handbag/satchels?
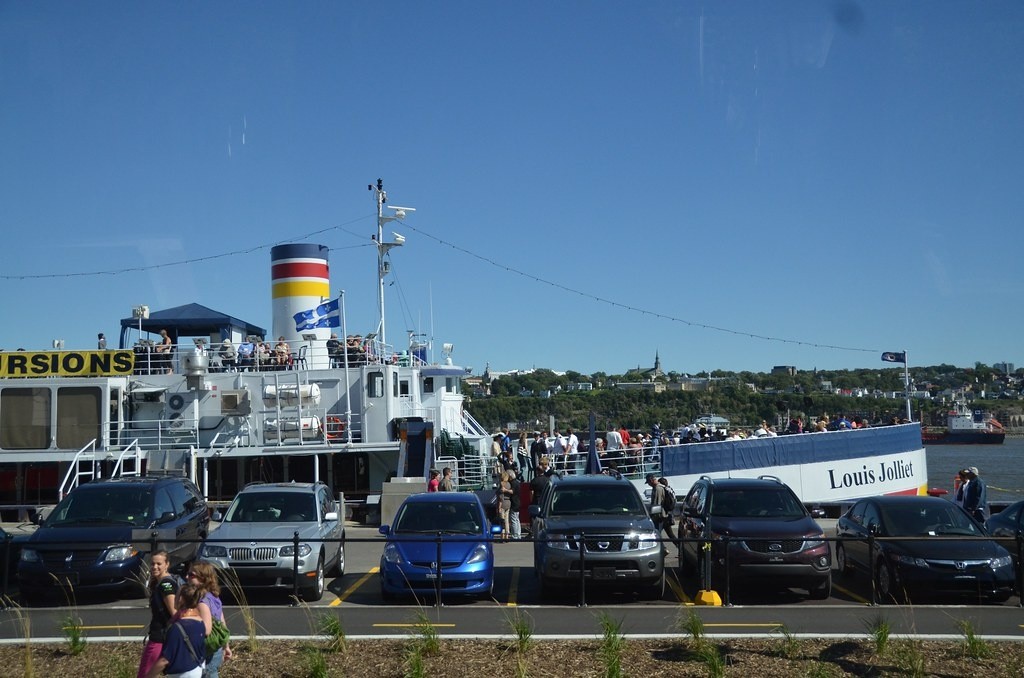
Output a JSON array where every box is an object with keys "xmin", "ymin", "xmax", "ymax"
[
  {"xmin": 202, "ymin": 597, "xmax": 230, "ymax": 660},
  {"xmin": 975, "ymin": 505, "xmax": 990, "ymax": 522}
]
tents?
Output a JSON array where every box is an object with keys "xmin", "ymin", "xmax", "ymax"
[{"xmin": 118, "ymin": 302, "xmax": 267, "ymax": 349}]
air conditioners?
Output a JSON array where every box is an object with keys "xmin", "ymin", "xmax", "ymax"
[{"xmin": 166, "ymin": 392, "xmax": 200, "ymax": 431}]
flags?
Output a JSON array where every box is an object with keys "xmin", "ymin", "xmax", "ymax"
[
  {"xmin": 292, "ymin": 298, "xmax": 340, "ymax": 332},
  {"xmin": 881, "ymin": 352, "xmax": 905, "ymax": 363}
]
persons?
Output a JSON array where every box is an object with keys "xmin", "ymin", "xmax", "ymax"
[
  {"xmin": 98, "ymin": 333, "xmax": 106, "ymax": 349},
  {"xmin": 491, "ymin": 428, "xmax": 579, "ymax": 542},
  {"xmin": 645, "ymin": 473, "xmax": 679, "ymax": 556},
  {"xmin": 427, "ymin": 470, "xmax": 441, "ymax": 492},
  {"xmin": 951, "ymin": 466, "xmax": 987, "ymax": 524},
  {"xmin": 580, "ymin": 419, "xmax": 777, "ymax": 477},
  {"xmin": 788, "ymin": 413, "xmax": 871, "ymax": 434},
  {"xmin": 888, "ymin": 416, "xmax": 911, "ymax": 425},
  {"xmin": 137, "ymin": 550, "xmax": 232, "ymax": 678},
  {"xmin": 156, "ymin": 329, "xmax": 175, "ymax": 372},
  {"xmin": 438, "ymin": 468, "xmax": 453, "ymax": 491},
  {"xmin": 217, "ymin": 335, "xmax": 290, "ymax": 371},
  {"xmin": 327, "ymin": 334, "xmax": 387, "ymax": 369}
]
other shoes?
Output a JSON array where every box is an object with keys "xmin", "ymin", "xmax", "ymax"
[{"xmin": 664, "ymin": 549, "xmax": 670, "ymax": 557}]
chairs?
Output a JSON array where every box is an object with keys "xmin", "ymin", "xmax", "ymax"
[
  {"xmin": 557, "ymin": 493, "xmax": 576, "ymax": 509},
  {"xmin": 205, "ymin": 348, "xmax": 217, "ymax": 372},
  {"xmin": 328, "ymin": 347, "xmax": 343, "ymax": 368},
  {"xmin": 434, "ymin": 428, "xmax": 481, "ymax": 483},
  {"xmin": 452, "ymin": 511, "xmax": 474, "ymax": 523},
  {"xmin": 291, "ymin": 345, "xmax": 309, "ymax": 370},
  {"xmin": 250, "ymin": 501, "xmax": 275, "ymax": 520}
]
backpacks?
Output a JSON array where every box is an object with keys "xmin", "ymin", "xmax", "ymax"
[{"xmin": 654, "ymin": 485, "xmax": 676, "ymax": 515}]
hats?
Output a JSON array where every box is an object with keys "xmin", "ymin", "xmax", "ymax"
[
  {"xmin": 964, "ymin": 466, "xmax": 979, "ymax": 476},
  {"xmin": 637, "ymin": 434, "xmax": 644, "ymax": 438},
  {"xmin": 222, "ymin": 338, "xmax": 231, "ymax": 348},
  {"xmin": 645, "ymin": 473, "xmax": 655, "ymax": 484},
  {"xmin": 532, "ymin": 430, "xmax": 541, "ymax": 436}
]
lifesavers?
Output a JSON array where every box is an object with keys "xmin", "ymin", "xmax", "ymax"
[{"xmin": 321, "ymin": 417, "xmax": 344, "ymax": 438}]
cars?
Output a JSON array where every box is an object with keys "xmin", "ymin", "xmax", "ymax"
[
  {"xmin": 378, "ymin": 492, "xmax": 501, "ymax": 602},
  {"xmin": 839, "ymin": 495, "xmax": 1024, "ymax": 604}
]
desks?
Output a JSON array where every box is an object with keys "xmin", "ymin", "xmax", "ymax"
[{"xmin": 134, "ymin": 351, "xmax": 168, "ymax": 374}]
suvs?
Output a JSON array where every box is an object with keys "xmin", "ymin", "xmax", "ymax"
[
  {"xmin": 18, "ymin": 473, "xmax": 214, "ymax": 602},
  {"xmin": 680, "ymin": 475, "xmax": 833, "ymax": 598},
  {"xmin": 533, "ymin": 476, "xmax": 667, "ymax": 599},
  {"xmin": 196, "ymin": 485, "xmax": 348, "ymax": 601}
]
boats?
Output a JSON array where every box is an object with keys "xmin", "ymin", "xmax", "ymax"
[
  {"xmin": 1, "ymin": 176, "xmax": 930, "ymax": 520},
  {"xmin": 920, "ymin": 401, "xmax": 1009, "ymax": 446}
]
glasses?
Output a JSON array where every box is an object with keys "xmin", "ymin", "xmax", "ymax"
[{"xmin": 186, "ymin": 571, "xmax": 205, "ymax": 583}]
